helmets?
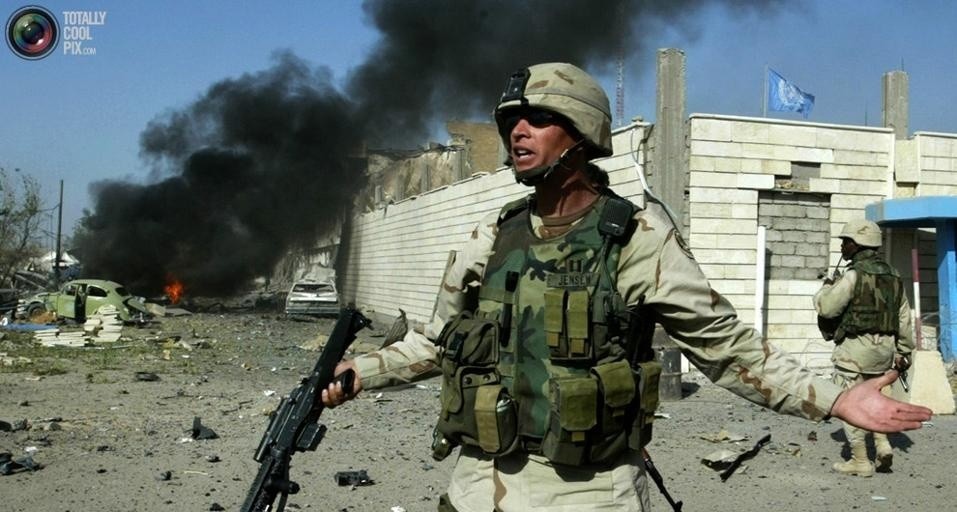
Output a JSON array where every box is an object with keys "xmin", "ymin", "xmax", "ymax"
[
  {"xmin": 837, "ymin": 219, "xmax": 883, "ymax": 247},
  {"xmin": 496, "ymin": 60, "xmax": 614, "ymax": 156}
]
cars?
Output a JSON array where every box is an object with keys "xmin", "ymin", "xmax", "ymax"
[
  {"xmin": 285, "ymin": 280, "xmax": 341, "ymax": 316},
  {"xmin": 14, "ymin": 279, "xmax": 154, "ymax": 324}
]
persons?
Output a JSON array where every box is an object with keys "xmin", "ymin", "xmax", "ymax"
[
  {"xmin": 813, "ymin": 218, "xmax": 913, "ymax": 478},
  {"xmin": 322, "ymin": 61, "xmax": 933, "ymax": 512}
]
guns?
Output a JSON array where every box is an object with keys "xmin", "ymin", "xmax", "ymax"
[
  {"xmin": 239, "ymin": 304, "xmax": 375, "ymax": 512},
  {"xmin": 642, "ymin": 447, "xmax": 683, "ymax": 512},
  {"xmin": 894, "ymin": 355, "xmax": 912, "ymax": 391}
]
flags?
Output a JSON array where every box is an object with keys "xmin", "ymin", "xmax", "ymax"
[{"xmin": 767, "ymin": 67, "xmax": 815, "ymax": 119}]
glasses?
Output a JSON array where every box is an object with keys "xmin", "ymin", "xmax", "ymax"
[{"xmin": 499, "ymin": 110, "xmax": 561, "ymax": 130}]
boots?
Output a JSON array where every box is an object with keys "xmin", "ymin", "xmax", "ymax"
[
  {"xmin": 874, "ymin": 430, "xmax": 894, "ymax": 473},
  {"xmin": 832, "ymin": 434, "xmax": 875, "ymax": 478}
]
